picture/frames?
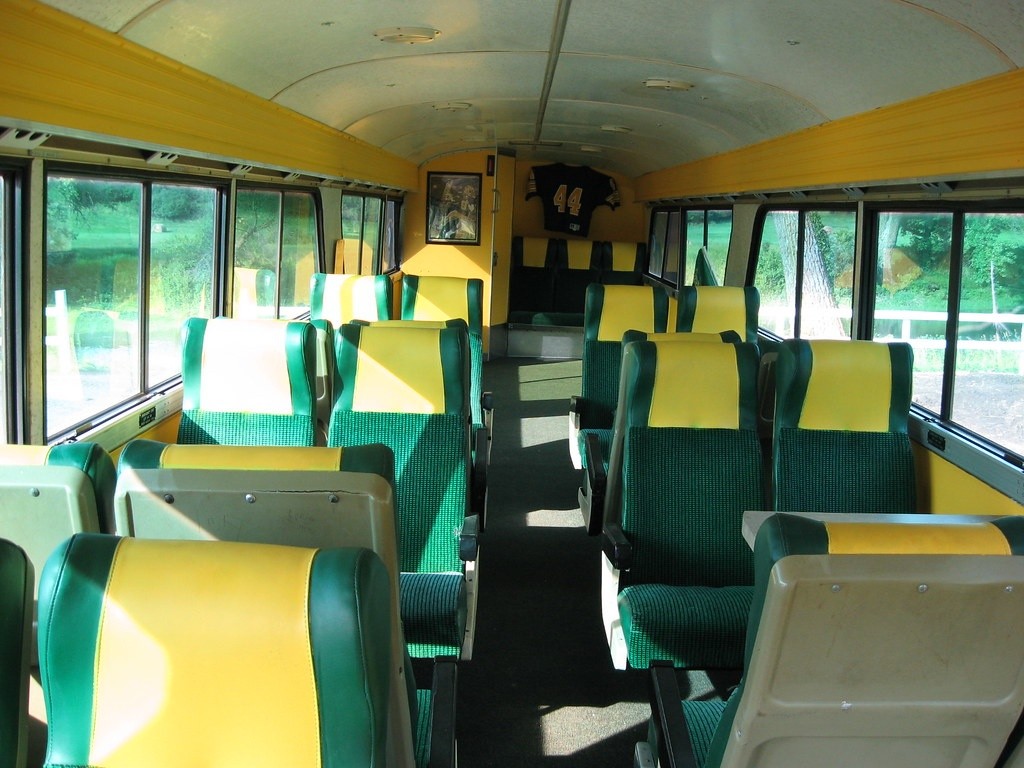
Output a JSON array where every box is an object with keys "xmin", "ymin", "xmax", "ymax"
[{"xmin": 426, "ymin": 171, "xmax": 482, "ymax": 246}]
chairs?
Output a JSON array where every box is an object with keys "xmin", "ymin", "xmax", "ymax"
[{"xmin": 0, "ymin": 236, "xmax": 1024, "ymax": 768}]
